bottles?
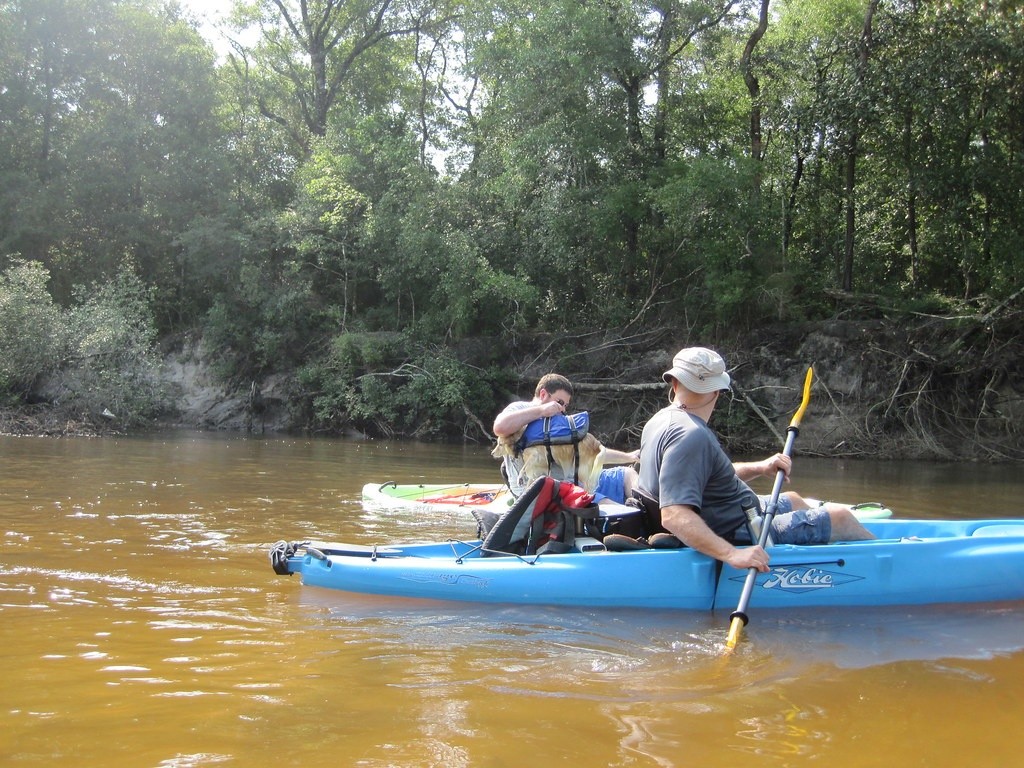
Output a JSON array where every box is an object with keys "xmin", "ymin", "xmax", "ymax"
[{"xmin": 745, "ymin": 507, "xmax": 773, "ymax": 546}]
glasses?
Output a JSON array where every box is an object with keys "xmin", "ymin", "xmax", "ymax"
[{"xmin": 549, "ymin": 393, "xmax": 568, "ymax": 408}]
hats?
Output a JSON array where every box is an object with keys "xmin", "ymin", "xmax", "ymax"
[{"xmin": 662, "ymin": 347, "xmax": 730, "ymax": 393}]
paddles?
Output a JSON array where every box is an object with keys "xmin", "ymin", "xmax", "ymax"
[{"xmin": 726, "ymin": 364, "xmax": 814, "ymax": 645}]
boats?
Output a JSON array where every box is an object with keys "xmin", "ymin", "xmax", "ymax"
[
  {"xmin": 359, "ymin": 482, "xmax": 893, "ymax": 518},
  {"xmin": 267, "ymin": 517, "xmax": 1023, "ymax": 612}
]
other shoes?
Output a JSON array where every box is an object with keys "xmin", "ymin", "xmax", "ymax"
[
  {"xmin": 603, "ymin": 534, "xmax": 651, "ymax": 550},
  {"xmin": 648, "ymin": 533, "xmax": 683, "ymax": 548}
]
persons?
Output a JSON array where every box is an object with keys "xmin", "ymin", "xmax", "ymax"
[
  {"xmin": 637, "ymin": 346, "xmax": 877, "ymax": 572},
  {"xmin": 493, "ymin": 373, "xmax": 640, "ymax": 503}
]
